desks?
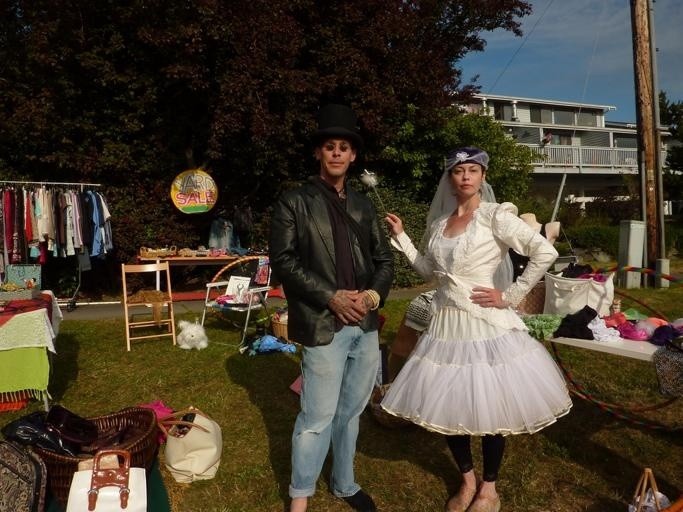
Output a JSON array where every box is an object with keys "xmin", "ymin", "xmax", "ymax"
[
  {"xmin": 135, "ymin": 247, "xmax": 268, "ymax": 308},
  {"xmin": 0, "ymin": 290, "xmax": 58, "ymax": 410},
  {"xmin": 520, "ymin": 310, "xmax": 682, "ymax": 371}
]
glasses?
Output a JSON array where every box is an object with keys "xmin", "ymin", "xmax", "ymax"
[{"xmin": 323, "ymin": 142, "xmax": 348, "ymax": 153}]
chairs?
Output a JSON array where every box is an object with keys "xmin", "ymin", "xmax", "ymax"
[
  {"xmin": 118, "ymin": 261, "xmax": 177, "ymax": 352},
  {"xmin": 200, "ymin": 254, "xmax": 272, "ymax": 347}
]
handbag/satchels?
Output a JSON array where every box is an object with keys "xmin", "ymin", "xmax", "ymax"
[
  {"xmin": 544, "ymin": 269, "xmax": 615, "ymax": 319},
  {"xmin": 164, "ymin": 405, "xmax": 222, "ymax": 484},
  {"xmin": 65, "ymin": 450, "xmax": 148, "ymax": 512}
]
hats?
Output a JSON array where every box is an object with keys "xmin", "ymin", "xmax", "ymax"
[{"xmin": 301, "ymin": 103, "xmax": 363, "ymax": 149}]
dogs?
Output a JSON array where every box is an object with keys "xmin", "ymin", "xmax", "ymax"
[{"xmin": 176, "ymin": 320, "xmax": 208, "ymax": 351}]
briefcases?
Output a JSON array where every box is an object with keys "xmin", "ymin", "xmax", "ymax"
[{"xmin": 0, "ymin": 263, "xmax": 42, "ymax": 301}]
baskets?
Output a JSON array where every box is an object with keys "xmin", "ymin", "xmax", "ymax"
[
  {"xmin": 515, "ymin": 280, "xmax": 545, "ymax": 315},
  {"xmin": 271, "ymin": 310, "xmax": 302, "ymax": 346},
  {"xmin": 6, "ymin": 408, "xmax": 158, "ymax": 512},
  {"xmin": 140, "ymin": 245, "xmax": 177, "ymax": 258},
  {"xmin": 368, "ymin": 384, "xmax": 413, "ymax": 427}
]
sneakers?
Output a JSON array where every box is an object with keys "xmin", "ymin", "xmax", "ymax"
[
  {"xmin": 445, "ymin": 481, "xmax": 479, "ymax": 511},
  {"xmin": 467, "ymin": 483, "xmax": 501, "ymax": 512}
]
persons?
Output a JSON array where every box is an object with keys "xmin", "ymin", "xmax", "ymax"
[
  {"xmin": 268, "ymin": 136, "xmax": 395, "ymax": 512},
  {"xmin": 379, "ymin": 146, "xmax": 573, "ymax": 512}
]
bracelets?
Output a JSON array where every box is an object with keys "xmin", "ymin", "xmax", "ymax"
[{"xmin": 365, "ymin": 289, "xmax": 381, "ymax": 311}]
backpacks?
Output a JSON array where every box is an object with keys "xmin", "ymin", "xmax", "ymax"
[{"xmin": 0, "ymin": 438, "xmax": 48, "ymax": 512}]
